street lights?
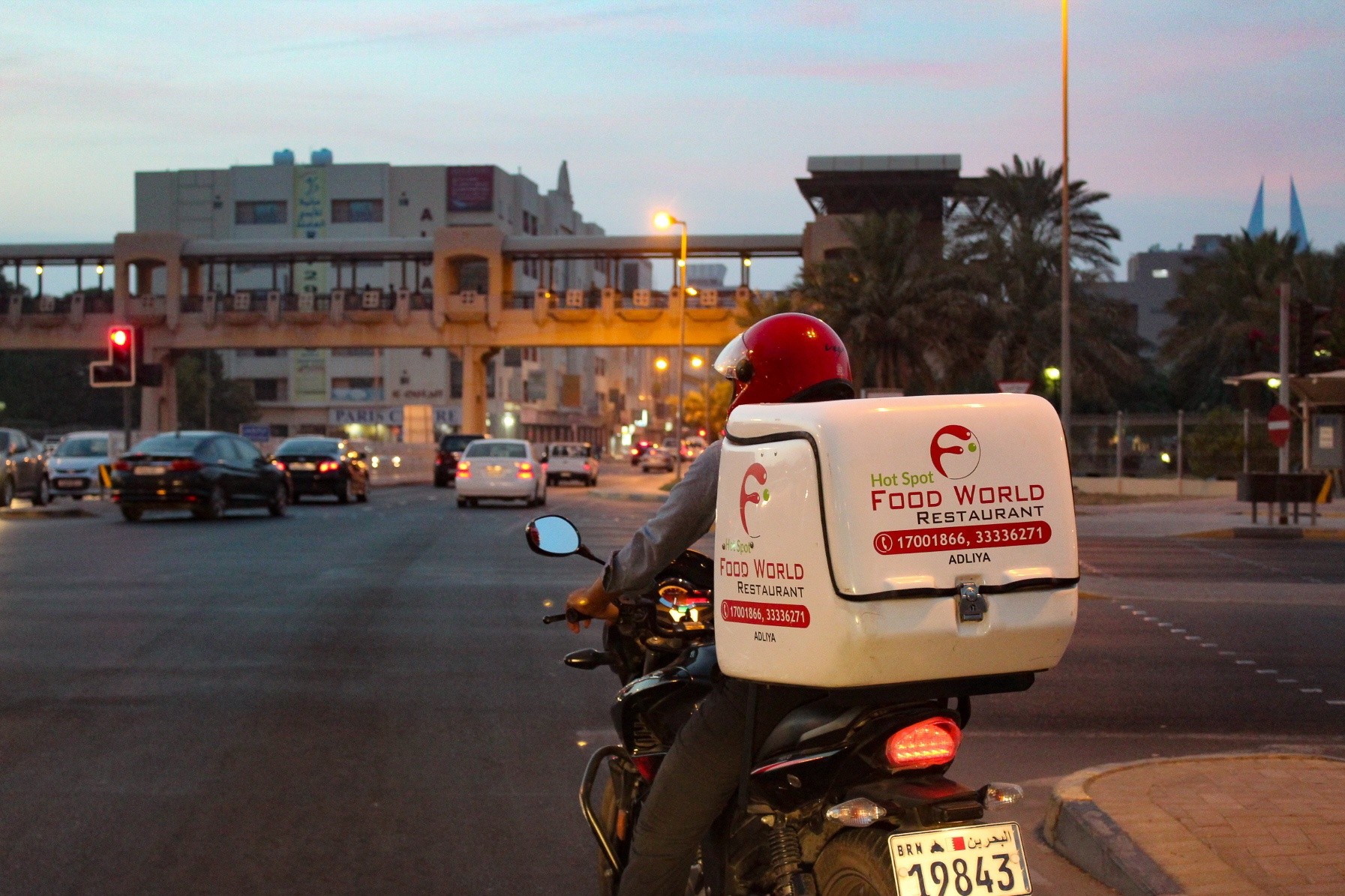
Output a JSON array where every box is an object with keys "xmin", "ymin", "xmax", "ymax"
[{"xmin": 654, "ymin": 213, "xmax": 682, "ymax": 483}]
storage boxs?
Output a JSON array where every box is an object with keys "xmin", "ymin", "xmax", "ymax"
[{"xmin": 709, "ymin": 395, "xmax": 1081, "ymax": 689}]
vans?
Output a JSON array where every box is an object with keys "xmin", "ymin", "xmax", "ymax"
[
  {"xmin": 433, "ymin": 434, "xmax": 486, "ymax": 488},
  {"xmin": 541, "ymin": 442, "xmax": 600, "ymax": 488}
]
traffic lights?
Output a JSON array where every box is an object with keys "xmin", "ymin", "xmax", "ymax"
[{"xmin": 90, "ymin": 325, "xmax": 135, "ymax": 388}]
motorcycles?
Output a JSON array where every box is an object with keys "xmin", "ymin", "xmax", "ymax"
[{"xmin": 519, "ymin": 387, "xmax": 1082, "ymax": 896}]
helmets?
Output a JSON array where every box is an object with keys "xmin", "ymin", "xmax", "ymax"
[{"xmin": 713, "ymin": 311, "xmax": 856, "ymax": 424}]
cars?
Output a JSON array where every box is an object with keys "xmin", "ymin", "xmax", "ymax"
[
  {"xmin": 43, "ymin": 432, "xmax": 122, "ymax": 502},
  {"xmin": 110, "ymin": 430, "xmax": 294, "ymax": 520},
  {"xmin": 451, "ymin": 438, "xmax": 550, "ymax": 508},
  {"xmin": 630, "ymin": 435, "xmax": 707, "ymax": 474},
  {"xmin": 272, "ymin": 435, "xmax": 370, "ymax": 506},
  {"xmin": 0, "ymin": 428, "xmax": 49, "ymax": 510}
]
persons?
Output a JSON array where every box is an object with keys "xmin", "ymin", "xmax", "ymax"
[{"xmin": 560, "ymin": 311, "xmax": 884, "ymax": 896}]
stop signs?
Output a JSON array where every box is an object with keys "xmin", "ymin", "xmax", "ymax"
[{"xmin": 1266, "ymin": 404, "xmax": 1290, "ymax": 448}]
